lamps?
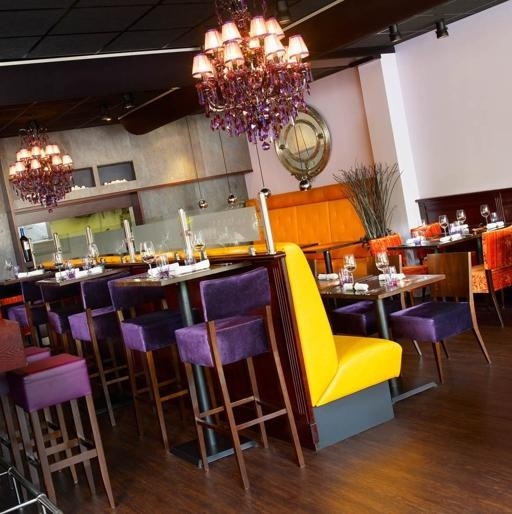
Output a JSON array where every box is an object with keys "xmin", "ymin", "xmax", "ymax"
[
  {"xmin": 190, "ymin": 1, "xmax": 316, "ymax": 156},
  {"xmin": 5, "ymin": 122, "xmax": 77, "ymax": 214}
]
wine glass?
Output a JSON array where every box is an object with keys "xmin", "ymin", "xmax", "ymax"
[
  {"xmin": 374, "ymin": 251, "xmax": 390, "ymax": 286},
  {"xmin": 439, "ymin": 205, "xmax": 490, "ymax": 238},
  {"xmin": 139, "ymin": 241, "xmax": 156, "ymax": 279},
  {"xmin": 344, "ymin": 254, "xmax": 357, "ymax": 283},
  {"xmin": 190, "ymin": 231, "xmax": 205, "ymax": 262},
  {"xmin": 52, "ymin": 251, "xmax": 64, "ymax": 281},
  {"xmin": 88, "ymin": 243, "xmax": 100, "ymax": 269}
]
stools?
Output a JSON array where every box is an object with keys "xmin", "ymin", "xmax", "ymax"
[
  {"xmin": 174, "ymin": 265, "xmax": 308, "ymax": 490},
  {"xmin": 1, "ymin": 343, "xmax": 79, "ymax": 501},
  {"xmin": 9, "ymin": 352, "xmax": 115, "ymax": 507},
  {"xmin": 1, "ymin": 267, "xmax": 156, "ymax": 428},
  {"xmin": 108, "ymin": 270, "xmax": 220, "ymax": 452}
]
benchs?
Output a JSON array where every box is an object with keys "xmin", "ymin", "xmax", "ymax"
[
  {"xmin": 41, "ymin": 240, "xmax": 402, "ymax": 452},
  {"xmin": 241, "ymin": 178, "xmax": 378, "ymax": 285}
]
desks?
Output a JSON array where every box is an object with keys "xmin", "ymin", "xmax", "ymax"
[{"xmin": 114, "ymin": 259, "xmax": 257, "ymax": 470}]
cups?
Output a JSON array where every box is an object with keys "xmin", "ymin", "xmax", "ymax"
[
  {"xmin": 383, "ymin": 266, "xmax": 400, "ymax": 292},
  {"xmin": 339, "ymin": 267, "xmax": 354, "ymax": 288},
  {"xmin": 156, "ymin": 255, "xmax": 168, "ymax": 274},
  {"xmin": 490, "ymin": 212, "xmax": 498, "ymax": 224},
  {"xmin": 64, "ymin": 256, "xmax": 93, "ymax": 278}
]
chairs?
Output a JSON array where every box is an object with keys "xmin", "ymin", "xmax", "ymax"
[{"xmin": 326, "ymin": 220, "xmax": 511, "ymax": 387}]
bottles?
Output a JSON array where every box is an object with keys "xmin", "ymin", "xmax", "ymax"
[{"xmin": 20, "ymin": 228, "xmax": 32, "ymax": 263}]
